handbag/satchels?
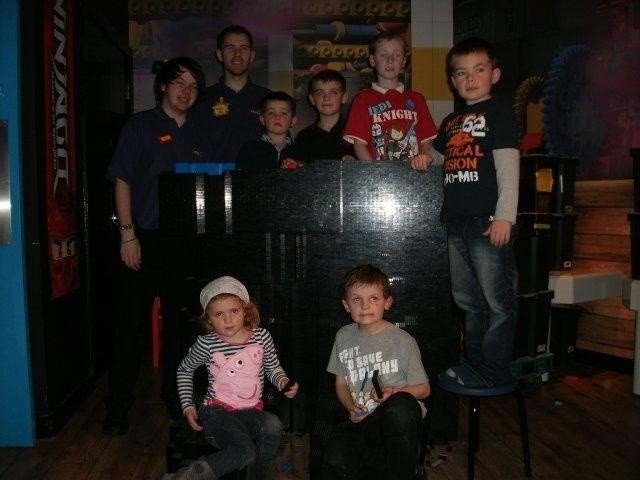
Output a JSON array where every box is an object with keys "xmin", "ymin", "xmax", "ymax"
[
  {"xmin": 119, "ymin": 223, "xmax": 137, "ymax": 232},
  {"xmin": 120, "ymin": 236, "xmax": 138, "ymax": 247}
]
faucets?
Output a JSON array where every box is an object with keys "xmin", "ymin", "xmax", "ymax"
[{"xmin": 443, "ymin": 361, "xmax": 505, "ymax": 387}]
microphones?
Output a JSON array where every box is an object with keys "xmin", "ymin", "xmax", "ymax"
[{"xmin": 423, "ymin": 365, "xmax": 533, "ymax": 480}]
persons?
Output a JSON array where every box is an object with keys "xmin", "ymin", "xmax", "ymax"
[
  {"xmin": 101, "ymin": 57, "xmax": 214, "ymax": 436},
  {"xmin": 325, "ymin": 264, "xmax": 432, "ymax": 480},
  {"xmin": 280, "ymin": 70, "xmax": 356, "ymax": 161},
  {"xmin": 339, "ymin": 31, "xmax": 439, "ymax": 161},
  {"xmin": 234, "ymin": 91, "xmax": 298, "ymax": 163},
  {"xmin": 170, "ymin": 275, "xmax": 299, "ymax": 480},
  {"xmin": 409, "ymin": 40, "xmax": 521, "ymax": 390},
  {"xmin": 188, "ymin": 25, "xmax": 272, "ymax": 163}
]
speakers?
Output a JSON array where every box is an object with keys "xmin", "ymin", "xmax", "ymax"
[
  {"xmin": 165, "ymin": 456, "xmax": 216, "ymax": 480},
  {"xmin": 252, "ymin": 459, "xmax": 281, "ymax": 478}
]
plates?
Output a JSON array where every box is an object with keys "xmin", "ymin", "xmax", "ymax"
[{"xmin": 504, "ymin": 151, "xmax": 580, "ymax": 382}]
